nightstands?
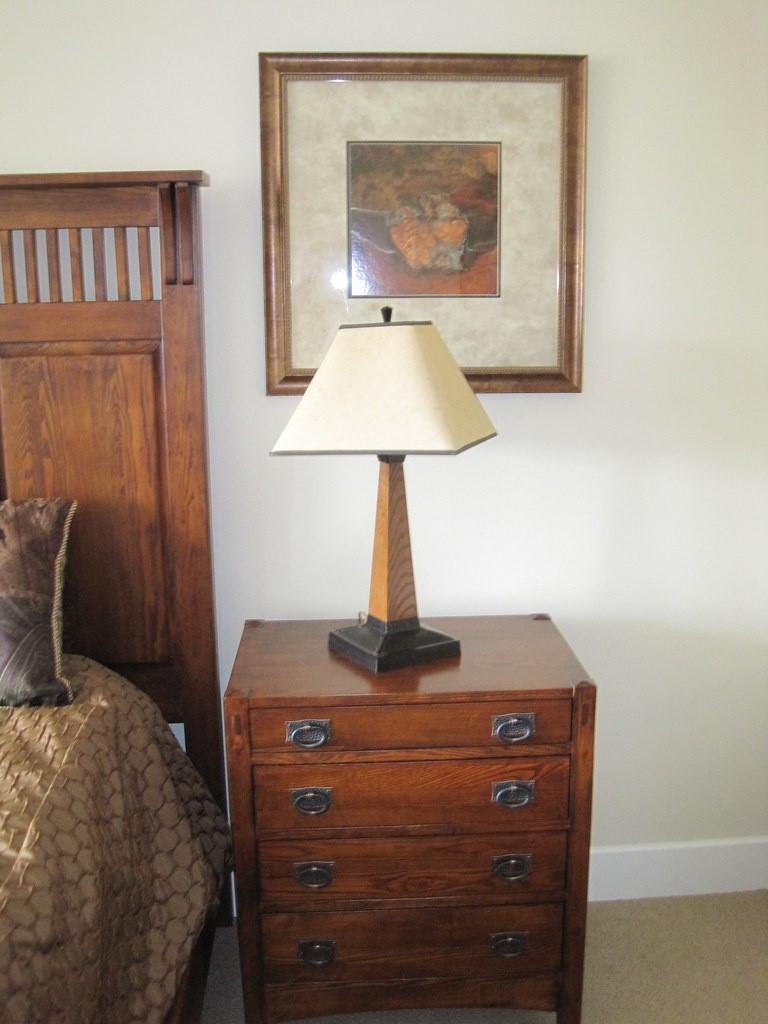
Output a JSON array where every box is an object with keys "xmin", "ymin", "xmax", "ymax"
[{"xmin": 223, "ymin": 614, "xmax": 598, "ymax": 1024}]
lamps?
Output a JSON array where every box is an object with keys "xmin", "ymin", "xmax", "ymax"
[{"xmin": 267, "ymin": 305, "xmax": 497, "ymax": 674}]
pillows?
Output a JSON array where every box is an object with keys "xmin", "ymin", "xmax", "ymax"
[{"xmin": 0, "ymin": 496, "xmax": 78, "ymax": 709}]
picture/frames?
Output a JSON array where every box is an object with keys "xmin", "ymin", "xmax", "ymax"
[{"xmin": 257, "ymin": 51, "xmax": 589, "ymax": 396}]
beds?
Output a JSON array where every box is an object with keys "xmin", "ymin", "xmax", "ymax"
[{"xmin": 0, "ymin": 170, "xmax": 234, "ymax": 1024}]
